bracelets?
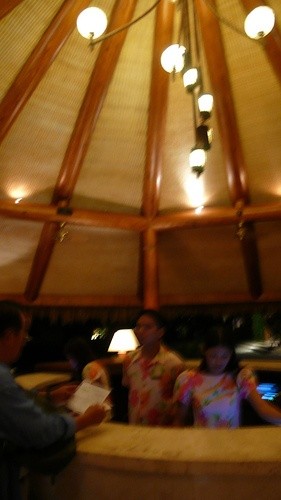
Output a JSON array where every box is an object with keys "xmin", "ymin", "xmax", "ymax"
[{"xmin": 44, "ymin": 388, "xmax": 54, "ymax": 409}]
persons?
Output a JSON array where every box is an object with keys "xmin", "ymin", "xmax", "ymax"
[
  {"xmin": 121, "ymin": 308, "xmax": 191, "ymax": 429},
  {"xmin": 0, "ymin": 298, "xmax": 108, "ymax": 500},
  {"xmin": 170, "ymin": 336, "xmax": 281, "ymax": 431}
]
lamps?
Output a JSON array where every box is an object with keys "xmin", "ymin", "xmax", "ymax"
[
  {"xmin": 106, "ymin": 328, "xmax": 140, "ymax": 356},
  {"xmin": 75, "ymin": 0, "xmax": 275, "ymax": 182}
]
